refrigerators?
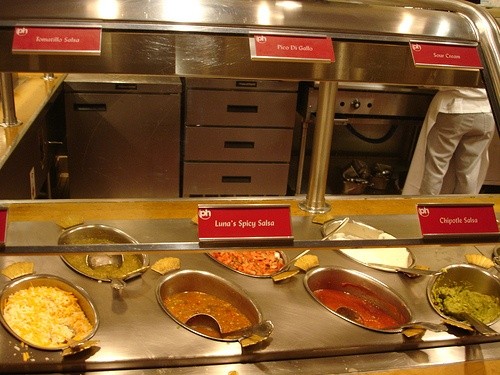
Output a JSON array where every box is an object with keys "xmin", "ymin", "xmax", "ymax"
[{"xmin": 62, "ymin": 71, "xmax": 179, "ymax": 198}]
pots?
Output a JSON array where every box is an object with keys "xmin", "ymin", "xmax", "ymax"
[
  {"xmin": 205, "ymin": 248, "xmax": 289, "ymax": 277},
  {"xmin": 0, "ymin": 273, "xmax": 99, "ymax": 351},
  {"xmin": 154, "ymin": 268, "xmax": 262, "ymax": 342},
  {"xmin": 59, "ymin": 224, "xmax": 149, "ymax": 282},
  {"xmin": 321, "ymin": 217, "xmax": 415, "ymax": 274},
  {"xmin": 426, "ymin": 263, "xmax": 500, "ymax": 328},
  {"xmin": 303, "ymin": 265, "xmax": 413, "ymax": 334}
]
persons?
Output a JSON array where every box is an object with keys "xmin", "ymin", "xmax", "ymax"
[{"xmin": 401, "ymin": 0, "xmax": 496, "ymax": 196}]
